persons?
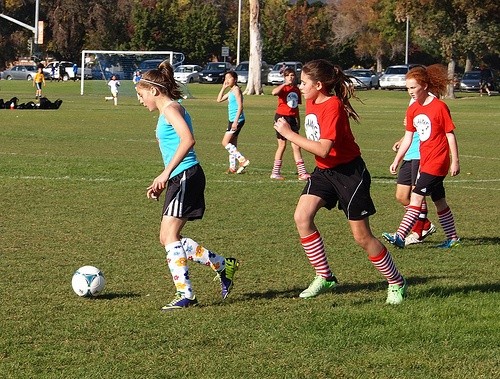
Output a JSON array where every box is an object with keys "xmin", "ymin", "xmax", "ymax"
[
  {"xmin": 133, "ymin": 70, "xmax": 142, "ymax": 84},
  {"xmin": 217, "ymin": 71, "xmax": 250, "ymax": 175},
  {"xmin": 381, "ymin": 63, "xmax": 461, "ymax": 248},
  {"xmin": 33, "ymin": 67, "xmax": 45, "ymax": 102},
  {"xmin": 136, "ymin": 60, "xmax": 241, "ymax": 309},
  {"xmin": 479, "ymin": 65, "xmax": 492, "ymax": 97},
  {"xmin": 393, "ymin": 64, "xmax": 436, "ymax": 245},
  {"xmin": 73, "ymin": 64, "xmax": 79, "ymax": 82},
  {"xmin": 272, "ymin": 68, "xmax": 312, "ymax": 181},
  {"xmin": 50, "ymin": 65, "xmax": 65, "ymax": 82},
  {"xmin": 105, "ymin": 75, "xmax": 120, "ymax": 106},
  {"xmin": 274, "ymin": 59, "xmax": 406, "ymax": 304}
]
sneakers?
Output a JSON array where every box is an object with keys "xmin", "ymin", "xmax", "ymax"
[
  {"xmin": 382, "ymin": 232, "xmax": 405, "ymax": 249},
  {"xmin": 213, "ymin": 258, "xmax": 240, "ymax": 300},
  {"xmin": 298, "ymin": 173, "xmax": 311, "ymax": 181},
  {"xmin": 161, "ymin": 292, "xmax": 199, "ymax": 310},
  {"xmin": 224, "ymin": 167, "xmax": 237, "ymax": 175},
  {"xmin": 422, "ymin": 223, "xmax": 437, "ymax": 239},
  {"xmin": 405, "ymin": 232, "xmax": 422, "ymax": 246},
  {"xmin": 386, "ymin": 276, "xmax": 408, "ymax": 305},
  {"xmin": 299, "ymin": 272, "xmax": 338, "ymax": 300},
  {"xmin": 270, "ymin": 173, "xmax": 285, "ymax": 180},
  {"xmin": 237, "ymin": 159, "xmax": 250, "ymax": 174},
  {"xmin": 436, "ymin": 237, "xmax": 461, "ymax": 249}
]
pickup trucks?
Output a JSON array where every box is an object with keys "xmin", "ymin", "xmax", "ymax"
[{"xmin": 42, "ymin": 60, "xmax": 93, "ymax": 80}]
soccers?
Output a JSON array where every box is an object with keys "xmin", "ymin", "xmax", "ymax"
[{"xmin": 71, "ymin": 265, "xmax": 107, "ymax": 297}]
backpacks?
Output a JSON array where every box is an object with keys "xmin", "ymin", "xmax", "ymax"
[{"xmin": 0, "ymin": 97, "xmax": 62, "ymax": 109}]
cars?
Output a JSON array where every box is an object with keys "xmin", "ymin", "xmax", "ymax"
[
  {"xmin": 378, "ymin": 64, "xmax": 428, "ymax": 90},
  {"xmin": 229, "ymin": 61, "xmax": 271, "ymax": 84},
  {"xmin": 266, "ymin": 61, "xmax": 305, "ymax": 85},
  {"xmin": 460, "ymin": 71, "xmax": 495, "ymax": 93},
  {"xmin": 198, "ymin": 62, "xmax": 233, "ymax": 84},
  {"xmin": 345, "ymin": 69, "xmax": 381, "ymax": 90},
  {"xmin": 2, "ymin": 64, "xmax": 51, "ymax": 82},
  {"xmin": 173, "ymin": 64, "xmax": 204, "ymax": 84},
  {"xmin": 131, "ymin": 59, "xmax": 165, "ymax": 80}
]
